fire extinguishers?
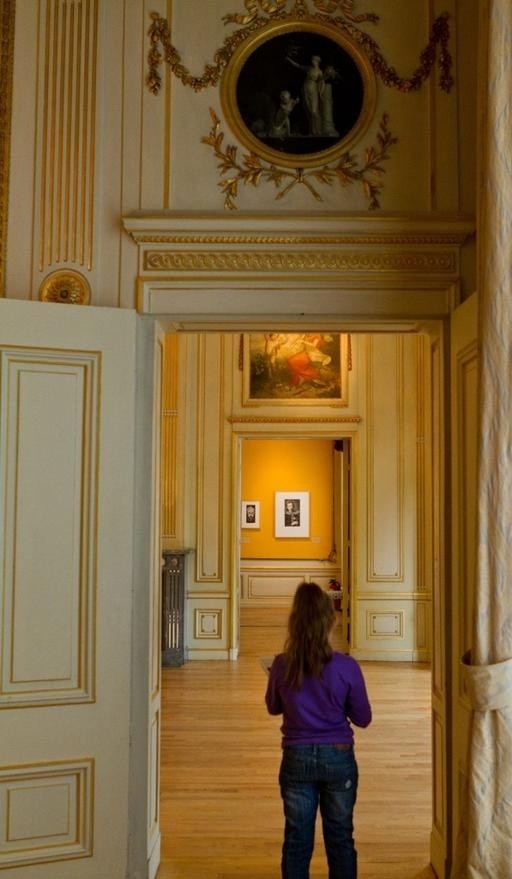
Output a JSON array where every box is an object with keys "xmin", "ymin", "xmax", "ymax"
[{"xmin": 328, "ymin": 579, "xmax": 341, "ymax": 612}]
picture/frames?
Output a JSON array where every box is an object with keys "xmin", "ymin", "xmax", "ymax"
[
  {"xmin": 274, "ymin": 491, "xmax": 310, "ymax": 538},
  {"xmin": 240, "ymin": 331, "xmax": 348, "ymax": 409},
  {"xmin": 240, "ymin": 501, "xmax": 260, "ymax": 529},
  {"xmin": 218, "ymin": 17, "xmax": 379, "ymax": 170}
]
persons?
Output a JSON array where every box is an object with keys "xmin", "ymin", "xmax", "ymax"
[
  {"xmin": 269, "ymin": 89, "xmax": 298, "ymax": 138},
  {"xmin": 286, "ymin": 53, "xmax": 326, "ymax": 135},
  {"xmin": 288, "ymin": 331, "xmax": 335, "ymax": 387},
  {"xmin": 285, "ymin": 502, "xmax": 299, "ymax": 526},
  {"xmin": 264, "ymin": 583, "xmax": 373, "ymax": 879},
  {"xmin": 263, "ymin": 333, "xmax": 286, "ymax": 384}
]
